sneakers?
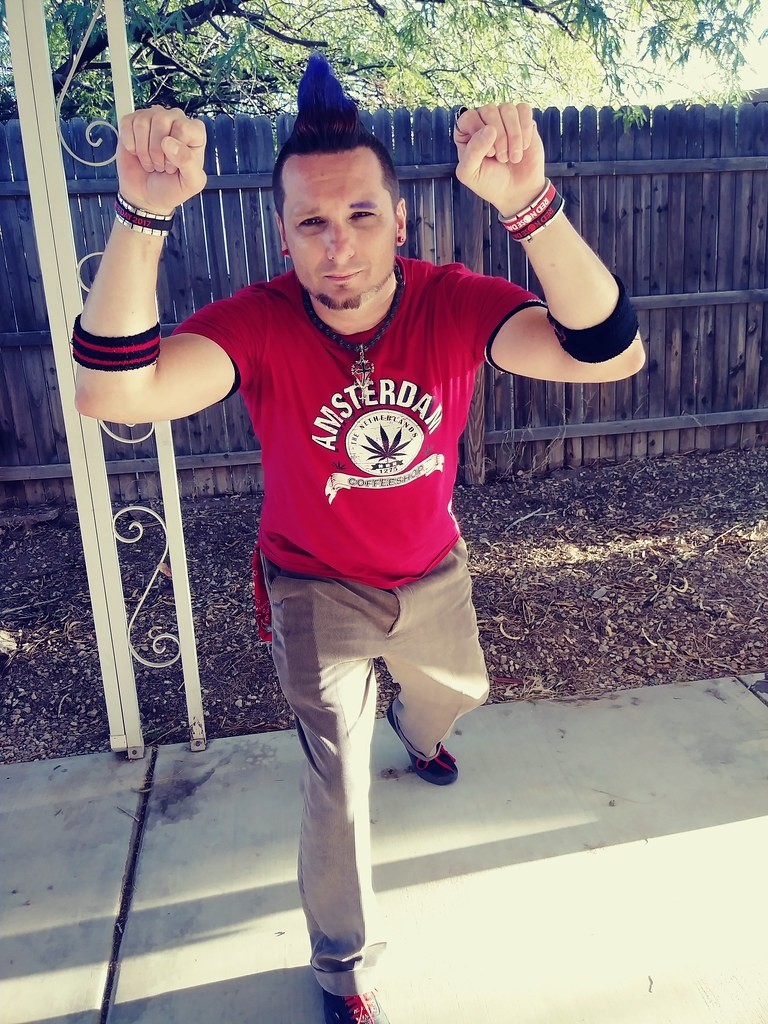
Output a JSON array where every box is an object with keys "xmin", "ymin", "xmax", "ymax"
[
  {"xmin": 387, "ymin": 705, "xmax": 458, "ymax": 785},
  {"xmin": 321, "ymin": 988, "xmax": 390, "ymax": 1024}
]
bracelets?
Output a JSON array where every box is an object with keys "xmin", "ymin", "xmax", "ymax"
[
  {"xmin": 70, "ymin": 312, "xmax": 161, "ymax": 372},
  {"xmin": 495, "ymin": 177, "xmax": 566, "ymax": 246},
  {"xmin": 111, "ymin": 191, "xmax": 178, "ymax": 241},
  {"xmin": 546, "ymin": 275, "xmax": 640, "ymax": 364}
]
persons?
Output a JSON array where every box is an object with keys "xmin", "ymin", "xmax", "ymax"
[{"xmin": 65, "ymin": 46, "xmax": 647, "ymax": 1023}]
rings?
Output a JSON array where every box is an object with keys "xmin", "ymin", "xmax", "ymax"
[{"xmin": 453, "ymin": 105, "xmax": 472, "ymax": 135}]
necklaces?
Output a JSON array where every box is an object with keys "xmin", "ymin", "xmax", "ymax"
[{"xmin": 300, "ymin": 260, "xmax": 403, "ymax": 390}]
